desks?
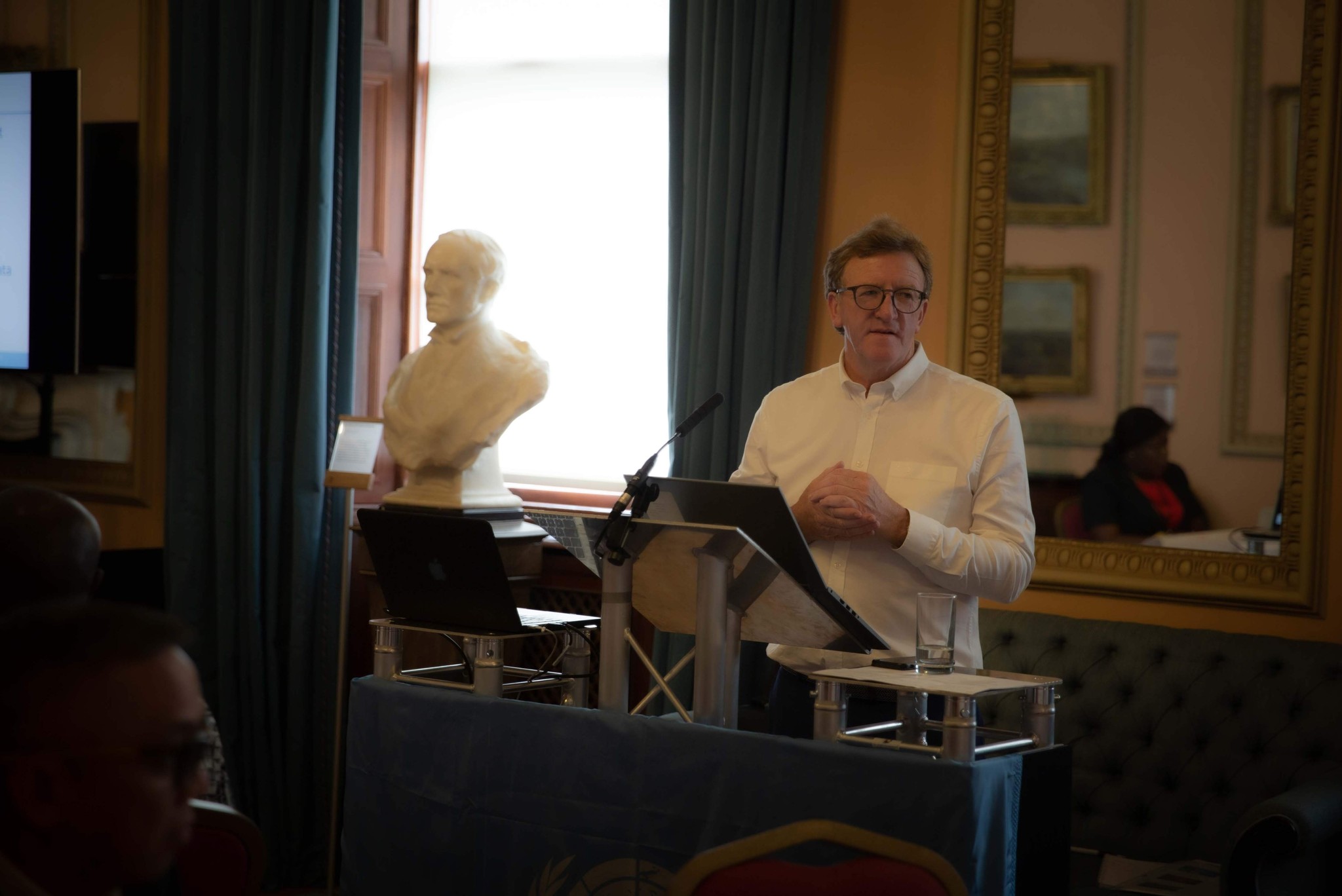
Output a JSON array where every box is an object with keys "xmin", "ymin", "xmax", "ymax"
[{"xmin": 340, "ymin": 676, "xmax": 1073, "ymax": 896}]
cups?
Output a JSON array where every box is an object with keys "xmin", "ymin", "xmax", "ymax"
[{"xmin": 916, "ymin": 593, "xmax": 958, "ymax": 675}]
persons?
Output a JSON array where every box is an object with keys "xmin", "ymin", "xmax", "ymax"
[
  {"xmin": 0, "ymin": 490, "xmax": 221, "ymax": 896},
  {"xmin": 381, "ymin": 229, "xmax": 549, "ymax": 512},
  {"xmin": 1080, "ymin": 405, "xmax": 1215, "ymax": 543},
  {"xmin": 728, "ymin": 214, "xmax": 1035, "ymax": 745}
]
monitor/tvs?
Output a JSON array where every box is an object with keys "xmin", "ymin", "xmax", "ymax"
[{"xmin": 0, "ymin": 68, "xmax": 85, "ymax": 374}]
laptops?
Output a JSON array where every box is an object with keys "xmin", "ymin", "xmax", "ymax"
[
  {"xmin": 356, "ymin": 508, "xmax": 601, "ymax": 635},
  {"xmin": 624, "ymin": 474, "xmax": 894, "ymax": 653},
  {"xmin": 1242, "ymin": 488, "xmax": 1284, "ymax": 540}
]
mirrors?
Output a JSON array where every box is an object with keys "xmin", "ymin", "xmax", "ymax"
[
  {"xmin": 952, "ymin": 0, "xmax": 1341, "ymax": 622},
  {"xmin": 43, "ymin": 1, "xmax": 162, "ymax": 508}
]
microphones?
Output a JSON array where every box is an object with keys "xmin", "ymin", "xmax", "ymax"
[{"xmin": 606, "ymin": 392, "xmax": 725, "ymax": 522}]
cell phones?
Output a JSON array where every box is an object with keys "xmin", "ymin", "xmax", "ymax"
[{"xmin": 871, "ymin": 656, "xmax": 917, "ymax": 670}]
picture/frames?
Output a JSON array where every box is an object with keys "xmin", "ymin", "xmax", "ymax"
[
  {"xmin": 998, "ymin": 266, "xmax": 1091, "ymax": 397},
  {"xmin": 1004, "ymin": 59, "xmax": 1112, "ymax": 227},
  {"xmin": 1267, "ymin": 84, "xmax": 1299, "ymax": 227}
]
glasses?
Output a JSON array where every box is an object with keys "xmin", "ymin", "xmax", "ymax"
[
  {"xmin": 835, "ymin": 285, "xmax": 928, "ymax": 313},
  {"xmin": 9, "ymin": 725, "xmax": 225, "ymax": 784}
]
